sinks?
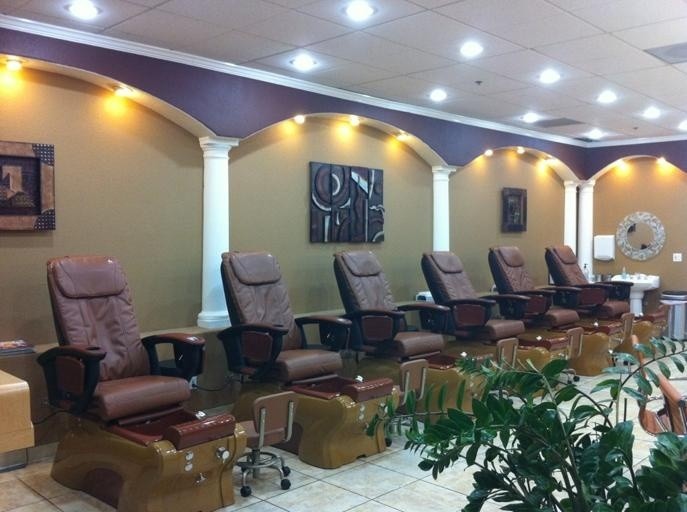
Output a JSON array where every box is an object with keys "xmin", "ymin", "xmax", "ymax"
[{"xmin": 610, "ymin": 274, "xmax": 660, "ymax": 291}]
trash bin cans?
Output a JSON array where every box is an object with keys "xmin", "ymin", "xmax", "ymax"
[{"xmin": 659, "ymin": 290, "xmax": 687, "ymax": 341}]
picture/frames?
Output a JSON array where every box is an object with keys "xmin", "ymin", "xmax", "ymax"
[
  {"xmin": 502, "ymin": 187, "xmax": 528, "ymax": 233},
  {"xmin": 0, "ymin": 141, "xmax": 56, "ymax": 231}
]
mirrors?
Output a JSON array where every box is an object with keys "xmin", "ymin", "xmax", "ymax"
[{"xmin": 616, "ymin": 212, "xmax": 666, "ymax": 261}]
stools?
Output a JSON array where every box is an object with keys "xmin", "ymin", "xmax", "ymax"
[
  {"xmin": 385, "ymin": 358, "xmax": 430, "ymax": 448},
  {"xmin": 556, "ymin": 327, "xmax": 584, "ymax": 385},
  {"xmin": 235, "ymin": 390, "xmax": 296, "ymax": 497}
]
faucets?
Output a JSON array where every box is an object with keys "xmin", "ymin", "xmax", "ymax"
[{"xmin": 635, "ymin": 272, "xmax": 640, "ymax": 280}]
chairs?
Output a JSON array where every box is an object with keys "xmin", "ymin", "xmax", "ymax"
[
  {"xmin": 216, "ymin": 250, "xmax": 399, "ymax": 470},
  {"xmin": 420, "ymin": 251, "xmax": 559, "ymax": 397},
  {"xmin": 39, "ymin": 254, "xmax": 248, "ymax": 512},
  {"xmin": 657, "ymin": 375, "xmax": 687, "ymax": 438},
  {"xmin": 487, "ymin": 246, "xmax": 668, "ymax": 378},
  {"xmin": 332, "ymin": 249, "xmax": 490, "ymax": 425}
]
